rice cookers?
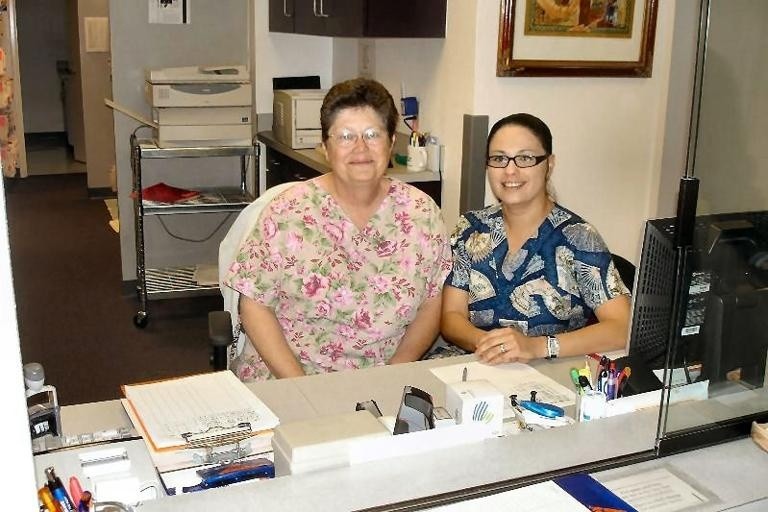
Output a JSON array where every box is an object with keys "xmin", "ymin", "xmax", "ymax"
[{"xmin": 196, "ymin": 459, "xmax": 276, "ymax": 490}]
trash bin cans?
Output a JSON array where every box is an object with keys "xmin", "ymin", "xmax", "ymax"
[{"xmin": 543, "ymin": 334, "xmax": 558, "ymax": 360}]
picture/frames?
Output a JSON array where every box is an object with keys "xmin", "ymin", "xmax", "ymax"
[{"xmin": 33, "ymin": 347, "xmax": 765, "ymax": 511}]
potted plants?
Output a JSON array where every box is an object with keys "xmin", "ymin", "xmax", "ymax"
[{"xmin": 494, "ymin": 0, "xmax": 659, "ymax": 80}]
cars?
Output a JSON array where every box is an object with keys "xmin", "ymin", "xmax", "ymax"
[{"xmin": 405, "ymin": 144, "xmax": 428, "ymax": 172}]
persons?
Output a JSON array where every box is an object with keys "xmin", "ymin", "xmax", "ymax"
[
  {"xmin": 224, "ymin": 76, "xmax": 452, "ymax": 383},
  {"xmin": 422, "ymin": 113, "xmax": 632, "ymax": 367}
]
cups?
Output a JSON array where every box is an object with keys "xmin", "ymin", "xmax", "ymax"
[
  {"xmin": 326, "ymin": 126, "xmax": 389, "ymax": 148},
  {"xmin": 485, "ymin": 144, "xmax": 549, "ymax": 169}
]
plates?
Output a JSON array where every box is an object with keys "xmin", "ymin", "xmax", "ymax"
[
  {"xmin": 258, "ymin": 128, "xmax": 440, "ymax": 215},
  {"xmin": 261, "ymin": 0, "xmax": 449, "ymax": 40}
]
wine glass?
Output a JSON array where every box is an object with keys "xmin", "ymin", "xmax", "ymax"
[{"xmin": 500, "ymin": 343, "xmax": 508, "ymax": 354}]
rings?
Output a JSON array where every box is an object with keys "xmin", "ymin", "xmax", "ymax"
[
  {"xmin": 462, "ymin": 368, "xmax": 468, "ymax": 382},
  {"xmin": 38, "ymin": 466, "xmax": 91, "ymax": 512},
  {"xmin": 571, "ymin": 356, "xmax": 631, "ymax": 402},
  {"xmin": 409, "ymin": 132, "xmax": 427, "ymax": 147}
]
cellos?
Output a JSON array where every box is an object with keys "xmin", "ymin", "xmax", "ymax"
[{"xmin": 273, "ymin": 75, "xmax": 329, "ymax": 150}]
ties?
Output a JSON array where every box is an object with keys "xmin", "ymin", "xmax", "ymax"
[{"xmin": 627, "ymin": 210, "xmax": 768, "ymax": 379}]
